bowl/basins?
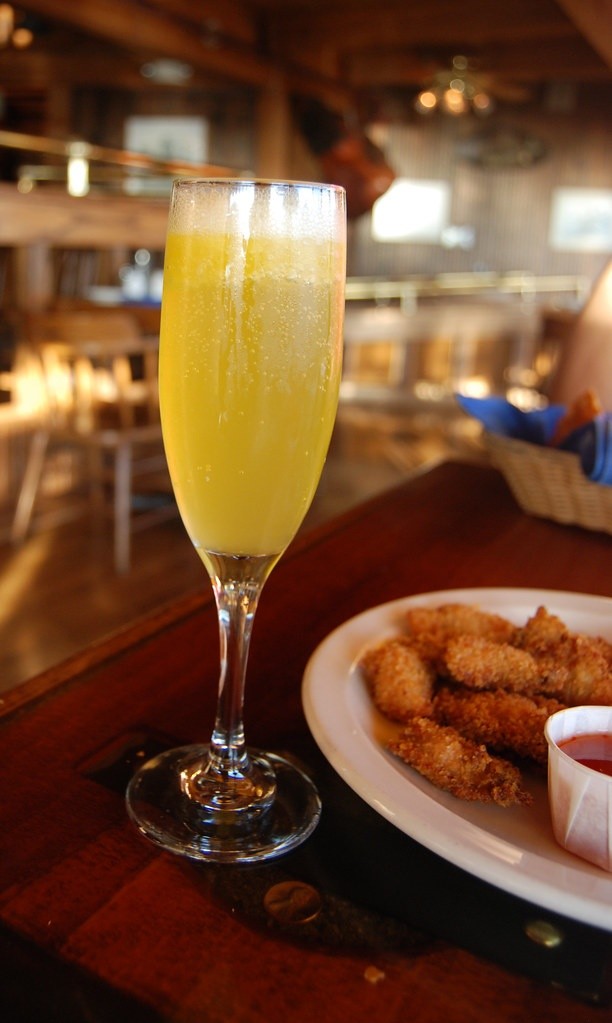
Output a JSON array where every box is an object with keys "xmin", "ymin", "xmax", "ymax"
[{"xmin": 544, "ymin": 705, "xmax": 612, "ymax": 874}]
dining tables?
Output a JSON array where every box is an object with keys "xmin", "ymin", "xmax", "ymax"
[{"xmin": 0, "ymin": 453, "xmax": 612, "ymax": 1022}]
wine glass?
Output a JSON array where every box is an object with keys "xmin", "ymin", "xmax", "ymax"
[{"xmin": 126, "ymin": 177, "xmax": 349, "ymax": 864}]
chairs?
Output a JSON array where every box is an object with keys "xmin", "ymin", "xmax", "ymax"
[{"xmin": 13, "ymin": 336, "xmax": 161, "ymax": 572}]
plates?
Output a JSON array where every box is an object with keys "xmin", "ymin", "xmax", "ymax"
[{"xmin": 301, "ymin": 586, "xmax": 612, "ymax": 933}]
